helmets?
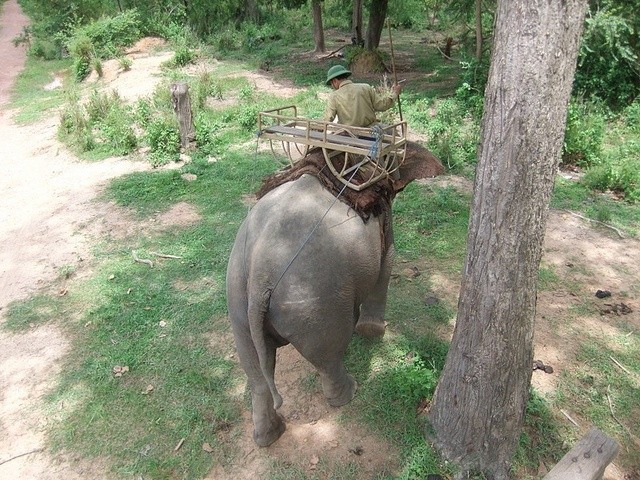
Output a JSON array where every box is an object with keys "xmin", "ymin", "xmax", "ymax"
[{"xmin": 325, "ymin": 65, "xmax": 352, "ymax": 85}]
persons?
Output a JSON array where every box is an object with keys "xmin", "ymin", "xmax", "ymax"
[{"xmin": 317, "ymin": 65, "xmax": 406, "ymax": 191}]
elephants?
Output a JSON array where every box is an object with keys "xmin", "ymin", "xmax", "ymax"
[{"xmin": 225, "ymin": 123, "xmax": 444, "ymax": 448}]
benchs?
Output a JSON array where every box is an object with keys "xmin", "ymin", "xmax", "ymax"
[{"xmin": 257, "ymin": 105, "xmax": 409, "ymax": 190}]
